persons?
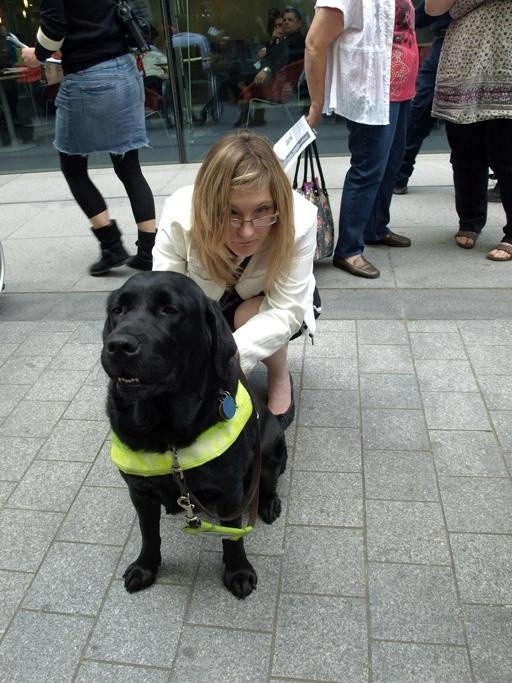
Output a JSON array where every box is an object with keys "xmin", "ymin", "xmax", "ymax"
[
  {"xmin": 303, "ymin": 0, "xmax": 420, "ymax": 280},
  {"xmin": 424, "ymin": 0, "xmax": 511, "ymax": 262},
  {"xmin": 232, "ymin": 13, "xmax": 287, "ymax": 129},
  {"xmin": 21, "ymin": 0, "xmax": 158, "ymax": 277},
  {"xmin": 152, "ymin": 131, "xmax": 320, "ymax": 433},
  {"xmin": 227, "ymin": 8, "xmax": 306, "ymax": 105},
  {"xmin": 139, "ymin": 24, "xmax": 170, "ymax": 97},
  {"xmin": 391, "ymin": 0, "xmax": 501, "ymax": 204}
]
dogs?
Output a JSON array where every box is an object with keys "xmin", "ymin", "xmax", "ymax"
[{"xmin": 101, "ymin": 270, "xmax": 295, "ymax": 598}]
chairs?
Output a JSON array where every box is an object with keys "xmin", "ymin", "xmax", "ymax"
[
  {"xmin": 237, "ymin": 59, "xmax": 304, "ymax": 129},
  {"xmin": 144, "ymin": 87, "xmax": 174, "ymax": 148}
]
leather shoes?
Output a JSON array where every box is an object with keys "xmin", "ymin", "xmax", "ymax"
[
  {"xmin": 334, "ymin": 254, "xmax": 380, "ymax": 279},
  {"xmin": 376, "ymin": 231, "xmax": 411, "ymax": 246},
  {"xmin": 266, "ymin": 371, "xmax": 294, "ymax": 431}
]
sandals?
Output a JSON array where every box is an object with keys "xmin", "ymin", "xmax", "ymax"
[
  {"xmin": 487, "ymin": 242, "xmax": 511, "ymax": 260},
  {"xmin": 455, "ymin": 230, "xmax": 479, "ymax": 249}
]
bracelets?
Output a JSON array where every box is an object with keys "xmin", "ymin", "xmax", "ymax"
[{"xmin": 262, "ymin": 66, "xmax": 271, "ymax": 73}]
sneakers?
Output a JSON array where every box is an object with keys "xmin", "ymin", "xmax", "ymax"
[
  {"xmin": 234, "ymin": 113, "xmax": 265, "ymax": 127},
  {"xmin": 392, "ymin": 176, "xmax": 409, "ymax": 193},
  {"xmin": 488, "ymin": 182, "xmax": 503, "ymax": 203}
]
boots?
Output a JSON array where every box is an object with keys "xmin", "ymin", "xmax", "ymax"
[
  {"xmin": 89, "ymin": 219, "xmax": 130, "ymax": 275},
  {"xmin": 126, "ymin": 230, "xmax": 159, "ymax": 271}
]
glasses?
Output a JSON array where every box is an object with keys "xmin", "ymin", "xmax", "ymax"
[{"xmin": 225, "ymin": 204, "xmax": 279, "ymax": 228}]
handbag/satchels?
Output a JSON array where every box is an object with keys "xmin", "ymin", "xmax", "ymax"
[
  {"xmin": 136, "ymin": 52, "xmax": 168, "ymax": 80},
  {"xmin": 291, "ymin": 175, "xmax": 335, "ymax": 263}
]
